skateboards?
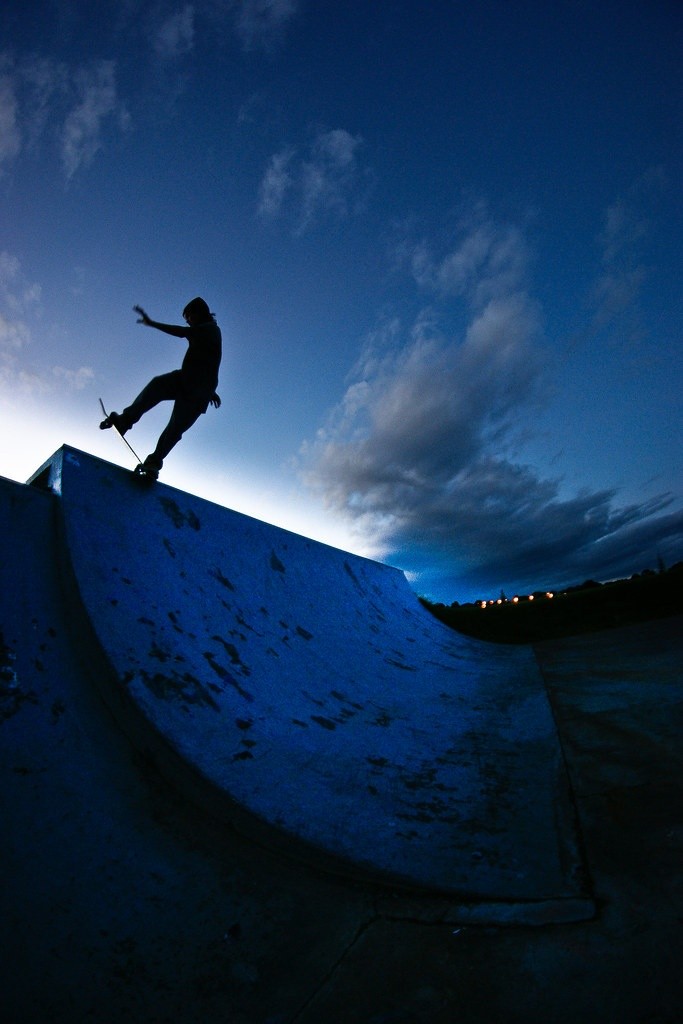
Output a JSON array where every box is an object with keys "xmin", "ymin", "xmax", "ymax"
[{"xmin": 98, "ymin": 397, "xmax": 158, "ymax": 485}]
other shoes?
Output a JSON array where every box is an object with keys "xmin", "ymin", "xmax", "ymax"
[
  {"xmin": 110, "ymin": 412, "xmax": 128, "ymax": 436},
  {"xmin": 144, "ymin": 455, "xmax": 159, "ymax": 479}
]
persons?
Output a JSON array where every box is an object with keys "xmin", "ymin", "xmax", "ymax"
[{"xmin": 101, "ymin": 298, "xmax": 224, "ymax": 484}]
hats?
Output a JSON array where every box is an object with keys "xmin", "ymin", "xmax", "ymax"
[{"xmin": 183, "ymin": 297, "xmax": 210, "ymax": 317}]
